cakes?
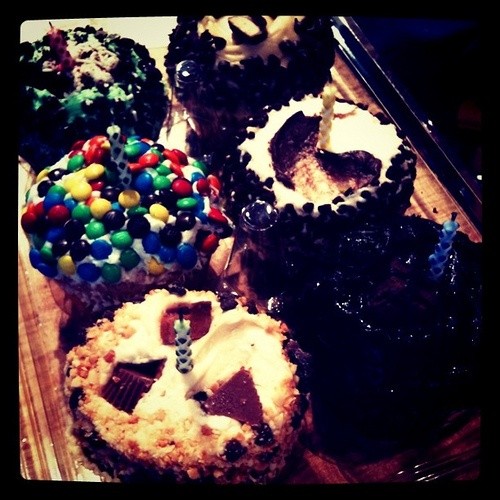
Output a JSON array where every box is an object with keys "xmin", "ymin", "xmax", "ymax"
[
  {"xmin": 17, "ymin": 23, "xmax": 168, "ymax": 173},
  {"xmin": 162, "ymin": 16, "xmax": 340, "ymax": 131},
  {"xmin": 262, "ymin": 216, "xmax": 495, "ymax": 460},
  {"xmin": 20, "ymin": 135, "xmax": 229, "ymax": 315},
  {"xmin": 64, "ymin": 285, "xmax": 313, "ymax": 488},
  {"xmin": 226, "ymin": 93, "xmax": 420, "ymax": 251}
]
